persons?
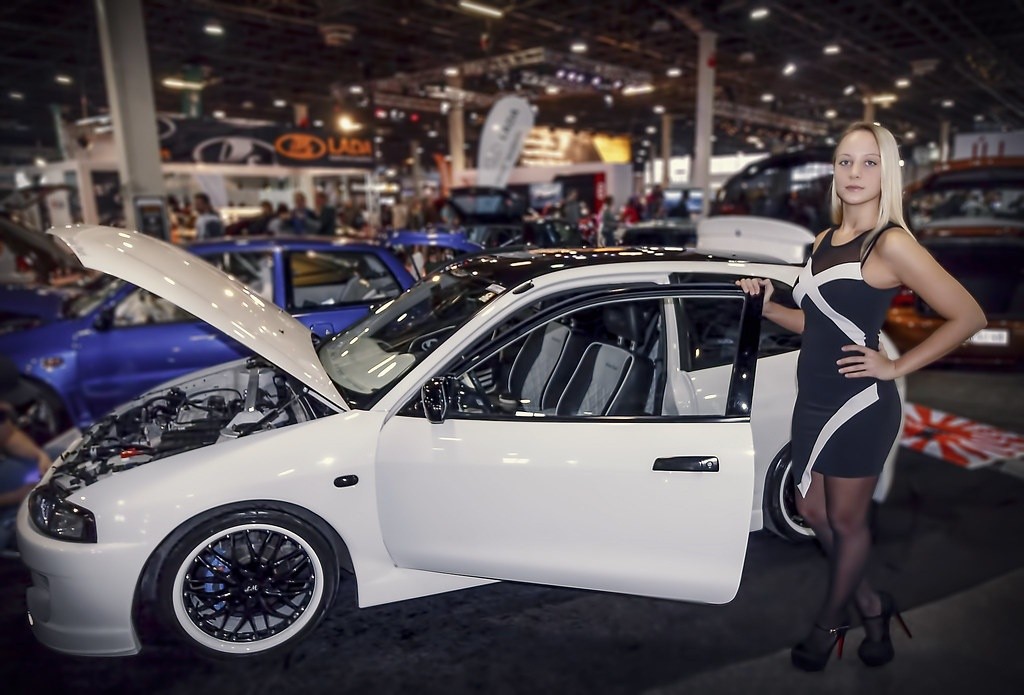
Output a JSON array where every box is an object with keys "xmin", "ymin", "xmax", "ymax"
[
  {"xmin": 559, "ymin": 184, "xmax": 691, "ymax": 247},
  {"xmin": 165, "ymin": 191, "xmax": 337, "ymax": 241},
  {"xmin": 733, "ymin": 117, "xmax": 987, "ymax": 672},
  {"xmin": 0, "ymin": 398, "xmax": 53, "ymax": 509}
]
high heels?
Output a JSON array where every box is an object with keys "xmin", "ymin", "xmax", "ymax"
[
  {"xmin": 859, "ymin": 589, "xmax": 912, "ymax": 666},
  {"xmin": 791, "ymin": 614, "xmax": 852, "ymax": 670}
]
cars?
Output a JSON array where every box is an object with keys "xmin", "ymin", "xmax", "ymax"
[{"xmin": 0, "ymin": 94, "xmax": 1024, "ymax": 670}]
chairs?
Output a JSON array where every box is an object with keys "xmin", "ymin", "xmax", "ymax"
[
  {"xmin": 555, "ymin": 302, "xmax": 653, "ymax": 416},
  {"xmin": 500, "ymin": 313, "xmax": 585, "ymax": 411}
]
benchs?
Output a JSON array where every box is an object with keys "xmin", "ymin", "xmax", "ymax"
[{"xmin": 342, "ymin": 277, "xmax": 388, "ymax": 300}]
